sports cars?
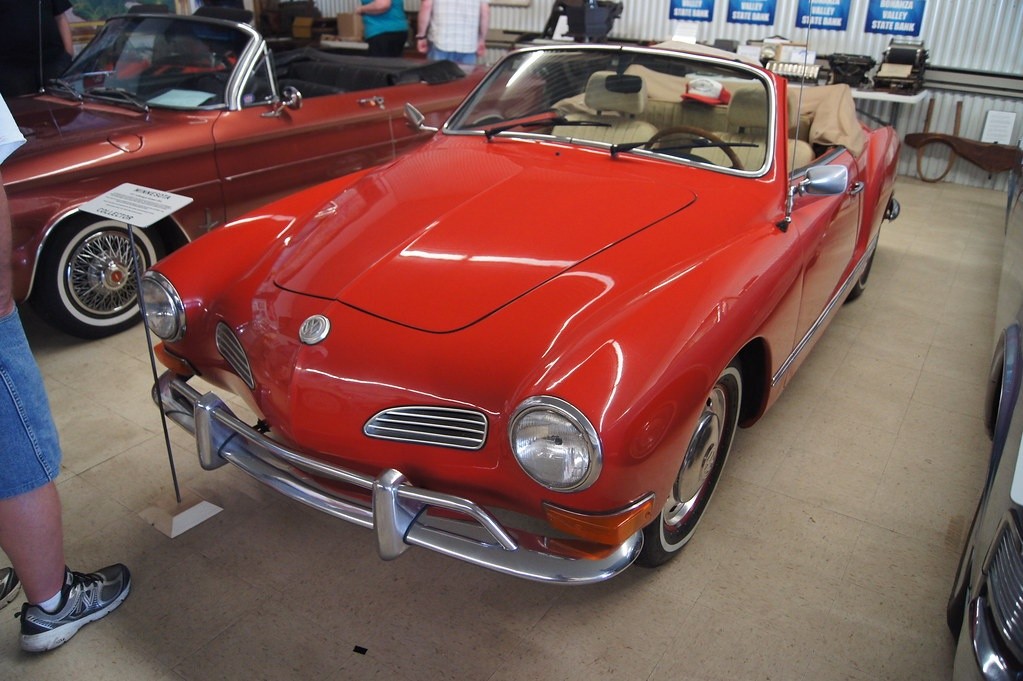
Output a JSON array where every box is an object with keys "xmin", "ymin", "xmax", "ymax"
[
  {"xmin": 136, "ymin": 0, "xmax": 902, "ymax": 586},
  {"xmin": 0, "ymin": 0, "xmax": 488, "ymax": 338}
]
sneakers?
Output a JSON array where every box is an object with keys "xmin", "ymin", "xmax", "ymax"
[
  {"xmin": 0, "ymin": 567, "xmax": 22, "ymax": 611},
  {"xmin": 15, "ymin": 563, "xmax": 130, "ymax": 651}
]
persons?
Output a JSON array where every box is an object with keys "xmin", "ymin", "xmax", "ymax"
[
  {"xmin": 355, "ymin": 0, "xmax": 411, "ymax": 58},
  {"xmin": 0, "ymin": 0, "xmax": 78, "ymax": 96},
  {"xmin": 0, "ymin": 76, "xmax": 132, "ymax": 655},
  {"xmin": 415, "ymin": 1, "xmax": 491, "ymax": 68}
]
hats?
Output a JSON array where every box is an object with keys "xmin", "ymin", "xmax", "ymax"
[{"xmin": 681, "ymin": 77, "xmax": 731, "ymax": 105}]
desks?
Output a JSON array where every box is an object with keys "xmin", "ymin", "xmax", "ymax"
[{"xmin": 849, "ymin": 90, "xmax": 929, "ymax": 127}]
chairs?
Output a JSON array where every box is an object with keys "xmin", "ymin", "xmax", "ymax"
[
  {"xmin": 551, "ymin": 71, "xmax": 660, "ymax": 151},
  {"xmin": 689, "ymin": 85, "xmax": 815, "ymax": 174}
]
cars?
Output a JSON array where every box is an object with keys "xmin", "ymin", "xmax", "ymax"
[{"xmin": 948, "ymin": 119, "xmax": 1023, "ymax": 681}]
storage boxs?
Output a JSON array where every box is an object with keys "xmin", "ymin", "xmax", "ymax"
[{"xmin": 336, "ymin": 12, "xmax": 363, "ymax": 39}]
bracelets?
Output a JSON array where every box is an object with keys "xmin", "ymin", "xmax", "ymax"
[{"xmin": 415, "ymin": 35, "xmax": 427, "ymax": 39}]
